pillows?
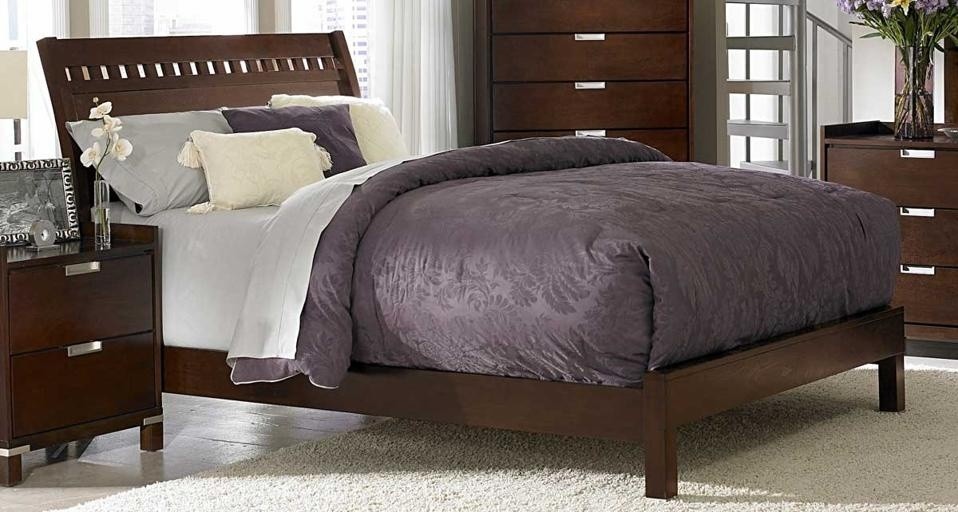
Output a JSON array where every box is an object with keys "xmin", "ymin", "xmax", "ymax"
[
  {"xmin": 220, "ymin": 102, "xmax": 268, "ymax": 111},
  {"xmin": 176, "ymin": 126, "xmax": 335, "ymax": 216},
  {"xmin": 63, "ymin": 110, "xmax": 230, "ymax": 220},
  {"xmin": 268, "ymin": 91, "xmax": 410, "ymax": 166},
  {"xmin": 223, "ymin": 104, "xmax": 368, "ymax": 180}
]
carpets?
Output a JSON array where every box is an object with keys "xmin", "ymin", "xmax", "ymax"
[{"xmin": 38, "ymin": 365, "xmax": 958, "ymax": 512}]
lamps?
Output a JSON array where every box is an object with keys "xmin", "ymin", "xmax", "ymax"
[{"xmin": 0, "ymin": 45, "xmax": 32, "ymax": 160}]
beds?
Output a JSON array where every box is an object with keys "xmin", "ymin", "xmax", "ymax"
[{"xmin": 34, "ymin": 29, "xmax": 909, "ymax": 498}]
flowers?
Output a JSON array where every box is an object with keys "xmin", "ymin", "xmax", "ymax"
[
  {"xmin": 833, "ymin": 0, "xmax": 958, "ymax": 139},
  {"xmin": 78, "ymin": 93, "xmax": 135, "ymax": 232}
]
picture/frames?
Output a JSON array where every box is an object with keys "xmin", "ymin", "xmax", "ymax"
[{"xmin": 1, "ymin": 157, "xmax": 80, "ymax": 248}]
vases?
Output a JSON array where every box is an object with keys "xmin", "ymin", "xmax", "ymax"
[
  {"xmin": 893, "ymin": 46, "xmax": 933, "ymax": 141},
  {"xmin": 91, "ymin": 179, "xmax": 112, "ymax": 248}
]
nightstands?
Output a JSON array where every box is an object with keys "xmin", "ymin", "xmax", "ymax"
[{"xmin": 0, "ymin": 221, "xmax": 169, "ymax": 488}]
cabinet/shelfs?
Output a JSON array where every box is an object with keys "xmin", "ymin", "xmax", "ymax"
[
  {"xmin": 820, "ymin": 120, "xmax": 958, "ymax": 343},
  {"xmin": 469, "ymin": 2, "xmax": 696, "ymax": 161}
]
